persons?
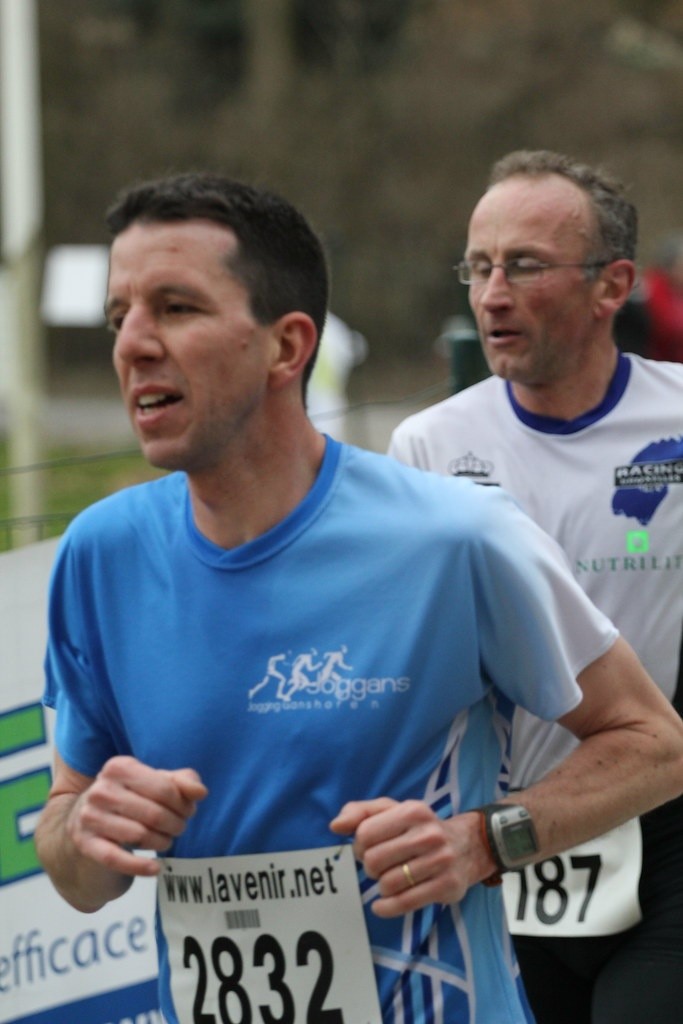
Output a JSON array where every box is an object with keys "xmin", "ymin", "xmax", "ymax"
[
  {"xmin": 385, "ymin": 149, "xmax": 683, "ymax": 1024},
  {"xmin": 30, "ymin": 172, "xmax": 683, "ymax": 1024},
  {"xmin": 642, "ymin": 235, "xmax": 682, "ymax": 363}
]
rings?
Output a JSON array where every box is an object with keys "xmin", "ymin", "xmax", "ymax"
[{"xmin": 402, "ymin": 864, "xmax": 416, "ymax": 888}]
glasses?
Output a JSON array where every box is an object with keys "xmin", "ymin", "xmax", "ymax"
[{"xmin": 452, "ymin": 255, "xmax": 606, "ymax": 285}]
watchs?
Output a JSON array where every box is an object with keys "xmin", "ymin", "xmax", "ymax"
[{"xmin": 467, "ymin": 804, "xmax": 540, "ymax": 888}]
